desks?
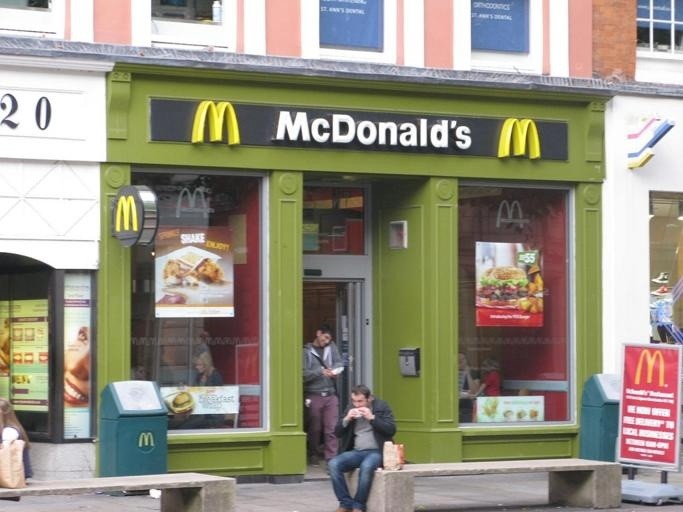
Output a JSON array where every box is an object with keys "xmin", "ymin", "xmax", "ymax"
[{"xmin": 459, "ymin": 394, "xmax": 476, "ymax": 400}]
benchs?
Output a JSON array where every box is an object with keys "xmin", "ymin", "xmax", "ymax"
[
  {"xmin": 341, "ymin": 458, "xmax": 622, "ymax": 512},
  {"xmin": 0, "ymin": 471, "xmax": 236, "ymax": 512}
]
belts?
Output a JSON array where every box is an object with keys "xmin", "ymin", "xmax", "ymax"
[{"xmin": 308, "ymin": 392, "xmax": 335, "ymax": 397}]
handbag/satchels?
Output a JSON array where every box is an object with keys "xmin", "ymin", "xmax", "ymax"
[{"xmin": 0, "ymin": 439, "xmax": 29, "ymax": 488}]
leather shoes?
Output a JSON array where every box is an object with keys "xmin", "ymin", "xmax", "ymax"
[{"xmin": 335, "ymin": 507, "xmax": 365, "ymax": 512}]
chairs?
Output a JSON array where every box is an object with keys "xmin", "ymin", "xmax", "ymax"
[{"xmin": 518, "ymin": 388, "xmax": 529, "ymax": 396}]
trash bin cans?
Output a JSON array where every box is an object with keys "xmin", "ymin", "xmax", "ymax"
[
  {"xmin": 579, "ymin": 373, "xmax": 623, "ymax": 462},
  {"xmin": 99, "ymin": 380, "xmax": 168, "ymax": 478}
]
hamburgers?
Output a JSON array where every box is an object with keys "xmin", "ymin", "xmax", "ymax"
[
  {"xmin": 170, "ymin": 391, "xmax": 193, "ymax": 413},
  {"xmin": 475, "ymin": 265, "xmax": 535, "ymax": 309}
]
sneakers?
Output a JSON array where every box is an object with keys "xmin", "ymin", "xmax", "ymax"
[
  {"xmin": 310, "ymin": 454, "xmax": 331, "ymax": 475},
  {"xmin": 651, "ymin": 272, "xmax": 672, "ymax": 309}
]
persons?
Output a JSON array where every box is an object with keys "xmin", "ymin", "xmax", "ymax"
[
  {"xmin": 0, "ymin": 398, "xmax": 33, "ymax": 485},
  {"xmin": 304, "ymin": 324, "xmax": 343, "ymax": 474},
  {"xmin": 458, "ymin": 352, "xmax": 480, "ymax": 394},
  {"xmin": 63, "ymin": 326, "xmax": 90, "ymax": 407},
  {"xmin": 179, "ymin": 352, "xmax": 224, "ymax": 429},
  {"xmin": 329, "ymin": 385, "xmax": 396, "ymax": 512},
  {"xmin": 132, "ymin": 364, "xmax": 146, "ymax": 379},
  {"xmin": 472, "ymin": 356, "xmax": 500, "ymax": 421}
]
